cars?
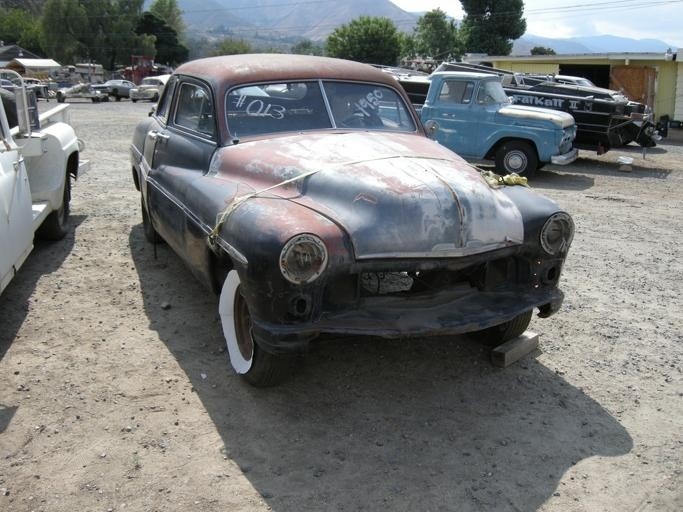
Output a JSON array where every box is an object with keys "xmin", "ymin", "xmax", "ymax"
[
  {"xmin": 126, "ymin": 48, "xmax": 577, "ymax": 388},
  {"xmin": 15, "ymin": 71, "xmax": 173, "ymax": 103}
]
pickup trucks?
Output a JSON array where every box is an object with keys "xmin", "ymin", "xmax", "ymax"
[
  {"xmin": 0, "ymin": 68, "xmax": 91, "ymax": 304},
  {"xmin": 370, "ymin": 69, "xmax": 578, "ymax": 181}
]
